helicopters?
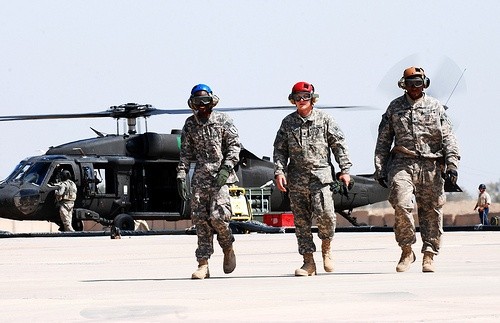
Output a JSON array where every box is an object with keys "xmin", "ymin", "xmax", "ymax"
[{"xmin": 0, "ymin": 102, "xmax": 463, "ymax": 231}]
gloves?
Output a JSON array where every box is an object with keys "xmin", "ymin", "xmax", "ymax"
[
  {"xmin": 178, "ymin": 179, "xmax": 189, "ymax": 201},
  {"xmin": 329, "ymin": 172, "xmax": 354, "ymax": 199},
  {"xmin": 213, "ymin": 165, "xmax": 230, "ymax": 187},
  {"xmin": 446, "ymin": 169, "xmax": 458, "ymax": 185},
  {"xmin": 377, "ymin": 174, "xmax": 388, "ymax": 188}
]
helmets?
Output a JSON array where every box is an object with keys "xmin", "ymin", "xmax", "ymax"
[
  {"xmin": 478, "ymin": 184, "xmax": 486, "ymax": 189},
  {"xmin": 403, "ymin": 67, "xmax": 425, "ymax": 78},
  {"xmin": 60, "ymin": 169, "xmax": 71, "ymax": 181},
  {"xmin": 191, "ymin": 83, "xmax": 212, "ymax": 95},
  {"xmin": 292, "ymin": 82, "xmax": 313, "ymax": 93}
]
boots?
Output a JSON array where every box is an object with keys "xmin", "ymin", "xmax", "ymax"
[
  {"xmin": 396, "ymin": 245, "xmax": 416, "ymax": 272},
  {"xmin": 321, "ymin": 239, "xmax": 334, "ymax": 273},
  {"xmin": 223, "ymin": 244, "xmax": 236, "ymax": 274},
  {"xmin": 422, "ymin": 252, "xmax": 435, "ymax": 272},
  {"xmin": 295, "ymin": 253, "xmax": 317, "ymax": 276},
  {"xmin": 192, "ymin": 259, "xmax": 210, "ymax": 279}
]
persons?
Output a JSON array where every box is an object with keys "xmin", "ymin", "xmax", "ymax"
[
  {"xmin": 54, "ymin": 171, "xmax": 78, "ymax": 231},
  {"xmin": 473, "ymin": 184, "xmax": 491, "ymax": 224},
  {"xmin": 174, "ymin": 84, "xmax": 241, "ymax": 279},
  {"xmin": 374, "ymin": 66, "xmax": 460, "ymax": 272},
  {"xmin": 273, "ymin": 82, "xmax": 352, "ymax": 275}
]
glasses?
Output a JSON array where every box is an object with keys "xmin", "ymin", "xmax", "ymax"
[
  {"xmin": 405, "ymin": 78, "xmax": 423, "ymax": 88},
  {"xmin": 191, "ymin": 96, "xmax": 212, "ymax": 106},
  {"xmin": 295, "ymin": 93, "xmax": 319, "ymax": 102}
]
